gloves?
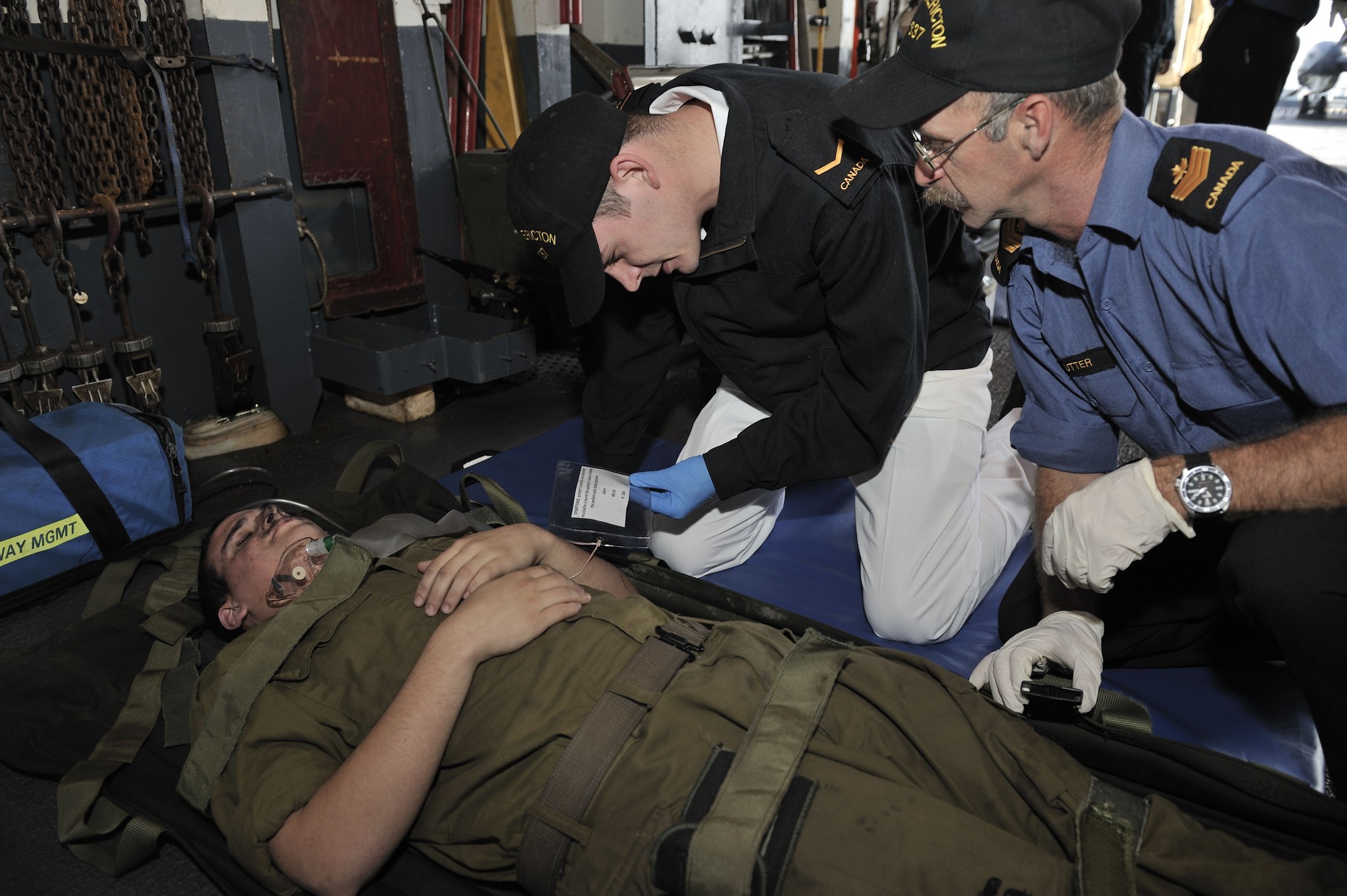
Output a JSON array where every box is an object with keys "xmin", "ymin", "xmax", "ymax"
[
  {"xmin": 967, "ymin": 611, "xmax": 1106, "ymax": 714},
  {"xmin": 1041, "ymin": 457, "xmax": 1197, "ymax": 594},
  {"xmin": 628, "ymin": 455, "xmax": 717, "ymax": 520}
]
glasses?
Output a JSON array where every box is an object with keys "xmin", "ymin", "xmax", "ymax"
[{"xmin": 912, "ymin": 94, "xmax": 1027, "ymax": 171}]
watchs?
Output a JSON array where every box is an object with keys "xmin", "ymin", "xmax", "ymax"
[{"xmin": 1174, "ymin": 451, "xmax": 1232, "ymax": 533}]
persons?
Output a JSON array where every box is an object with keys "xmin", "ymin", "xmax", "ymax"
[
  {"xmin": 912, "ymin": 0, "xmax": 1346, "ymax": 851},
  {"xmin": 508, "ymin": 62, "xmax": 1039, "ymax": 646},
  {"xmin": 1180, "ymin": 0, "xmax": 1322, "ymax": 132},
  {"xmin": 188, "ymin": 500, "xmax": 1347, "ymax": 895},
  {"xmin": 1116, "ymin": 0, "xmax": 1176, "ymax": 117}
]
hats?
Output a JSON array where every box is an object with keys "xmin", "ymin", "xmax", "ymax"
[
  {"xmin": 505, "ymin": 92, "xmax": 630, "ymax": 326},
  {"xmin": 829, "ymin": 0, "xmax": 1142, "ymax": 130}
]
